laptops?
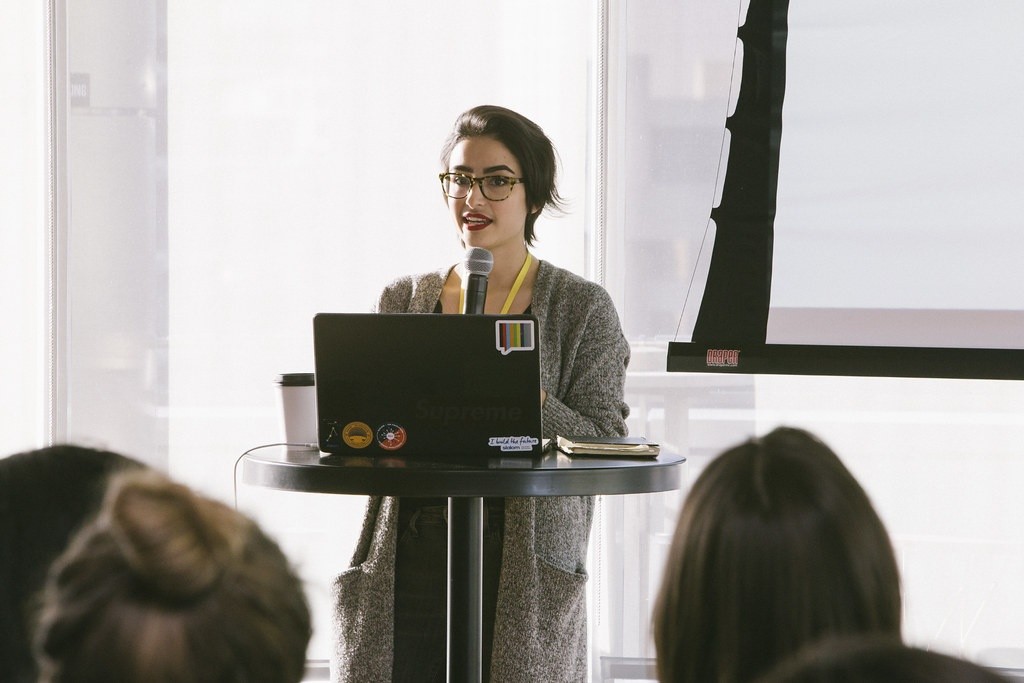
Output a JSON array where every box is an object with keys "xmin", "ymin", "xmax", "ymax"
[{"xmin": 313, "ymin": 314, "xmax": 551, "ymax": 457}]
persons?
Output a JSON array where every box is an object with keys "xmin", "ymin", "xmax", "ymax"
[
  {"xmin": 649, "ymin": 425, "xmax": 1018, "ymax": 683},
  {"xmin": 326, "ymin": 105, "xmax": 630, "ymax": 683},
  {"xmin": 0, "ymin": 442, "xmax": 311, "ymax": 683}
]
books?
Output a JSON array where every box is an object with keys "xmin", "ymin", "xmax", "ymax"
[{"xmin": 556, "ymin": 434, "xmax": 660, "ymax": 459}]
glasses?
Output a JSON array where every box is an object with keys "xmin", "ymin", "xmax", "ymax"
[{"xmin": 438, "ymin": 172, "xmax": 525, "ymax": 202}]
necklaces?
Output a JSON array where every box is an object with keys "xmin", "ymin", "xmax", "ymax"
[{"xmin": 459, "ymin": 248, "xmax": 531, "ymax": 317}]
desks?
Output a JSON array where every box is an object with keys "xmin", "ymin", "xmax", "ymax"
[{"xmin": 244, "ymin": 449, "xmax": 686, "ymax": 683}]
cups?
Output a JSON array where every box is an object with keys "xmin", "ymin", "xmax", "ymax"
[{"xmin": 274, "ymin": 372, "xmax": 317, "ymax": 451}]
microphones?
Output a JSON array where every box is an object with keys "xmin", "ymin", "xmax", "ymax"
[{"xmin": 462, "ymin": 247, "xmax": 493, "ymax": 314}]
